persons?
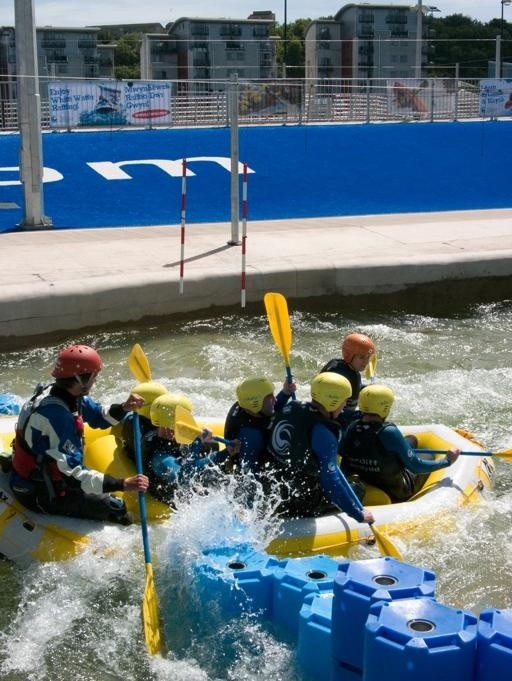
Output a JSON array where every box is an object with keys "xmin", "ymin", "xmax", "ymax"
[
  {"xmin": 143, "ymin": 392, "xmax": 241, "ymax": 510},
  {"xmin": 320, "ymin": 334, "xmax": 375, "ymax": 437},
  {"xmin": 247, "ymin": 372, "xmax": 375, "ymax": 524},
  {"xmin": 233, "ymin": 375, "xmax": 297, "ymax": 509},
  {"xmin": 341, "ymin": 384, "xmax": 461, "ymax": 503},
  {"xmin": 9, "ymin": 345, "xmax": 150, "ymax": 525},
  {"xmin": 121, "ymin": 382, "xmax": 168, "ymax": 466}
]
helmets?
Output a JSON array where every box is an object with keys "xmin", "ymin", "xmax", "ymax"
[
  {"xmin": 131, "ymin": 382, "xmax": 192, "ymax": 429},
  {"xmin": 343, "ymin": 334, "xmax": 376, "ymax": 363},
  {"xmin": 359, "ymin": 384, "xmax": 395, "ymax": 418},
  {"xmin": 51, "ymin": 345, "xmax": 104, "ymax": 378},
  {"xmin": 313, "ymin": 369, "xmax": 354, "ymax": 413},
  {"xmin": 237, "ymin": 376, "xmax": 275, "ymax": 413}
]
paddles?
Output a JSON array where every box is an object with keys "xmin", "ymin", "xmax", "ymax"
[
  {"xmin": 412, "ymin": 448, "xmax": 512, "ymax": 460},
  {"xmin": 134, "ymin": 412, "xmax": 161, "ymax": 654},
  {"xmin": 264, "ymin": 291, "xmax": 296, "ymax": 401},
  {"xmin": 127, "ymin": 344, "xmax": 150, "ymax": 382},
  {"xmin": 174, "ymin": 405, "xmax": 231, "ymax": 445},
  {"xmin": 337, "ymin": 467, "xmax": 402, "ymax": 561},
  {"xmin": 364, "ymin": 354, "xmax": 376, "ymax": 385}
]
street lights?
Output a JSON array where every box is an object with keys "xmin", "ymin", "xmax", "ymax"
[{"xmin": 501, "ymin": 0, "xmax": 512, "ymax": 77}]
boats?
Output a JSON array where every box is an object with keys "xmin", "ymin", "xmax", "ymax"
[{"xmin": 0, "ymin": 407, "xmax": 496, "ymax": 588}]
